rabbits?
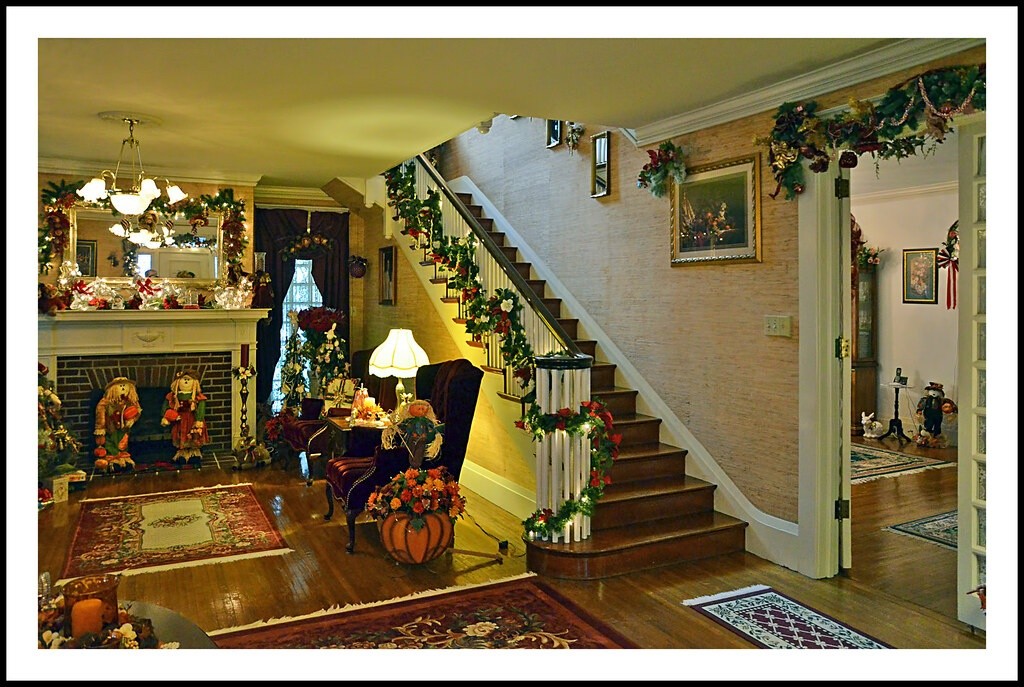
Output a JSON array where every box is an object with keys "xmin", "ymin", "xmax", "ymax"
[
  {"xmin": 111, "ymin": 297, "xmax": 125, "ymax": 309},
  {"xmin": 861, "ymin": 412, "xmax": 884, "ymax": 438}
]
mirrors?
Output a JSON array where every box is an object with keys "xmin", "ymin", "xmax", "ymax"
[{"xmin": 61, "ymin": 203, "xmax": 228, "ymax": 288}]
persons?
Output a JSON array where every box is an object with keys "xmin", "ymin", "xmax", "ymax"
[
  {"xmin": 397, "ymin": 400, "xmax": 445, "ymax": 469},
  {"xmin": 916, "ymin": 382, "xmax": 957, "ymax": 438},
  {"xmin": 160, "ymin": 369, "xmax": 208, "ymax": 470},
  {"xmin": 95, "ymin": 377, "xmax": 141, "ymax": 471}
]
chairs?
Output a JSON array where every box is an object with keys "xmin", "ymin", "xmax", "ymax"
[
  {"xmin": 324, "ymin": 360, "xmax": 485, "ymax": 557},
  {"xmin": 284, "ymin": 346, "xmax": 392, "ymax": 486}
]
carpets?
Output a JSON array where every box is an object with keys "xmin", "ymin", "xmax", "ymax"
[
  {"xmin": 852, "ymin": 440, "xmax": 957, "ymax": 485},
  {"xmin": 681, "ymin": 583, "xmax": 898, "ymax": 649},
  {"xmin": 882, "ymin": 508, "xmax": 957, "ymax": 551},
  {"xmin": 51, "ymin": 483, "xmax": 293, "ymax": 587}
]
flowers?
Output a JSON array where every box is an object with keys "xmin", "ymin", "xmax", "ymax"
[{"xmin": 38, "ymin": 115, "xmax": 968, "ymax": 538}]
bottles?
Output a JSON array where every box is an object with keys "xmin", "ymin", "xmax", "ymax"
[{"xmin": 60, "ymin": 572, "xmax": 121, "ymax": 639}]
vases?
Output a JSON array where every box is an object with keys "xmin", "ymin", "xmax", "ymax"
[{"xmin": 371, "ymin": 513, "xmax": 452, "ymax": 566}]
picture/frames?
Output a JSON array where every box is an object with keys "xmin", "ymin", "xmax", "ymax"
[
  {"xmin": 592, "ymin": 131, "xmax": 608, "ymax": 197},
  {"xmin": 544, "ymin": 119, "xmax": 561, "ymax": 147},
  {"xmin": 901, "ymin": 248, "xmax": 940, "ymax": 304},
  {"xmin": 379, "ymin": 246, "xmax": 396, "ymax": 306},
  {"xmin": 671, "ymin": 151, "xmax": 763, "ymax": 266}
]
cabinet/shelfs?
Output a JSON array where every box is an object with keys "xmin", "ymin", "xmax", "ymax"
[{"xmin": 854, "ymin": 265, "xmax": 877, "ymax": 437}]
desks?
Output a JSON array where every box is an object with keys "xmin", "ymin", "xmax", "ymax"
[
  {"xmin": 153, "ymin": 570, "xmax": 644, "ymax": 649},
  {"xmin": 326, "ymin": 416, "xmax": 383, "ymax": 458},
  {"xmin": 38, "ymin": 596, "xmax": 219, "ymax": 649}
]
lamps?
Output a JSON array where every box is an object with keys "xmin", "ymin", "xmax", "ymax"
[
  {"xmin": 109, "ymin": 212, "xmax": 176, "ymax": 249},
  {"xmin": 76, "ymin": 112, "xmax": 190, "ymax": 215},
  {"xmin": 368, "ymin": 328, "xmax": 429, "ymax": 414}
]
